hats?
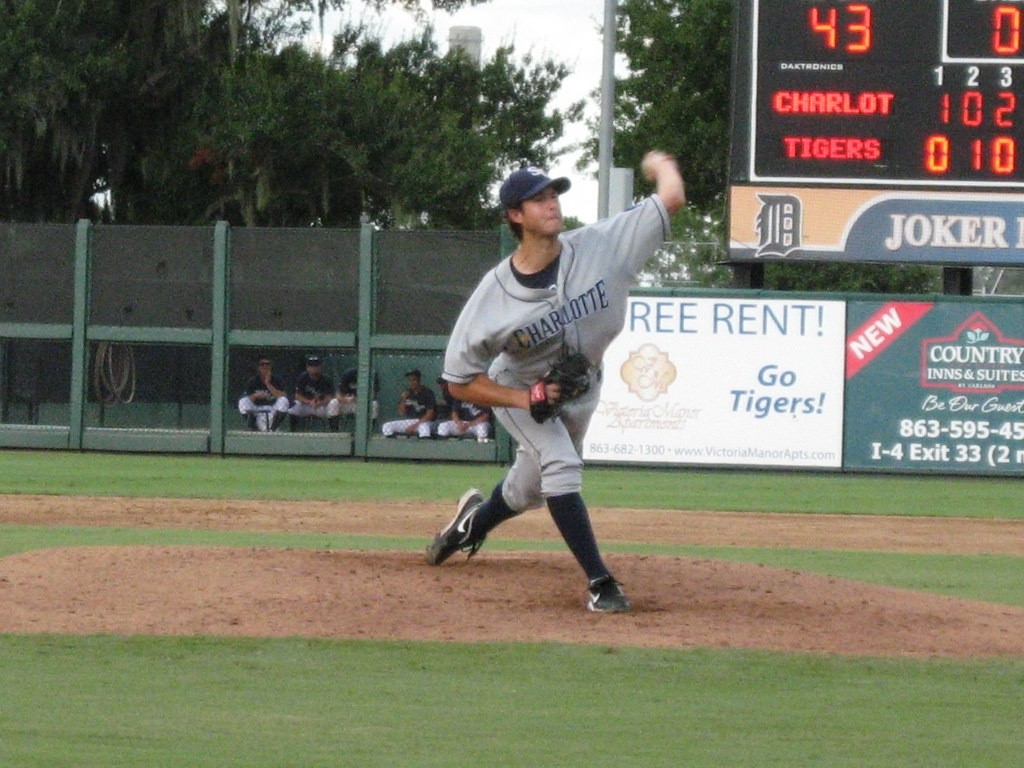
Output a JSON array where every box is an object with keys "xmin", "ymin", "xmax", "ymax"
[
  {"xmin": 500, "ymin": 170, "xmax": 571, "ymax": 208},
  {"xmin": 305, "ymin": 355, "xmax": 321, "ymax": 365},
  {"xmin": 405, "ymin": 368, "xmax": 421, "ymax": 377},
  {"xmin": 435, "ymin": 375, "xmax": 448, "ymax": 384}
]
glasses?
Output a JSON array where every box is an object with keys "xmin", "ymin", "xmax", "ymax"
[{"xmin": 257, "ymin": 362, "xmax": 270, "ymax": 367}]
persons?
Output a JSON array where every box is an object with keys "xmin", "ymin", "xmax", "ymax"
[
  {"xmin": 239, "ymin": 352, "xmax": 495, "ymax": 440},
  {"xmin": 426, "ymin": 151, "xmax": 685, "ymax": 612}
]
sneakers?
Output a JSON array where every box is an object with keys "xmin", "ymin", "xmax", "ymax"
[
  {"xmin": 583, "ymin": 575, "xmax": 630, "ymax": 614},
  {"xmin": 426, "ymin": 489, "xmax": 485, "ymax": 566}
]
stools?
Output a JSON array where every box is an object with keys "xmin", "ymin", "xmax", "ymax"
[{"xmin": 245, "ymin": 408, "xmax": 272, "ymax": 433}]
